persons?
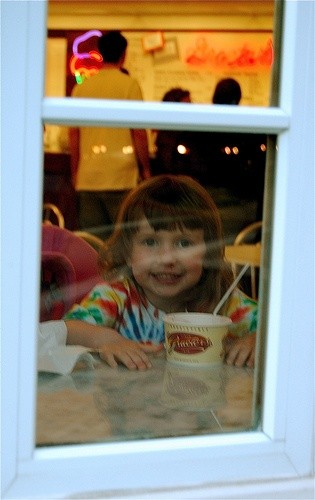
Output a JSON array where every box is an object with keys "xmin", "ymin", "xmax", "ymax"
[
  {"xmin": 199, "ymin": 78, "xmax": 266, "ymax": 187},
  {"xmin": 61, "ymin": 175, "xmax": 258, "ymax": 368},
  {"xmin": 69, "ymin": 31, "xmax": 152, "ymax": 246},
  {"xmin": 153, "ymin": 89, "xmax": 198, "ymax": 175}
]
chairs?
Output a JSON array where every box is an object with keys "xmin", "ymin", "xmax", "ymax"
[
  {"xmin": 39, "ymin": 200, "xmax": 111, "ymax": 324},
  {"xmin": 224, "ymin": 218, "xmax": 268, "ymax": 302}
]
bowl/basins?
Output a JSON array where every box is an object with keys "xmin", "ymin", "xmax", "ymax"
[
  {"xmin": 161, "ymin": 311, "xmax": 232, "ymax": 368},
  {"xmin": 159, "ymin": 362, "xmax": 228, "ymax": 412}
]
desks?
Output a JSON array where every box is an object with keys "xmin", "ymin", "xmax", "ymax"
[{"xmin": 36, "ymin": 356, "xmax": 263, "ymax": 446}]
